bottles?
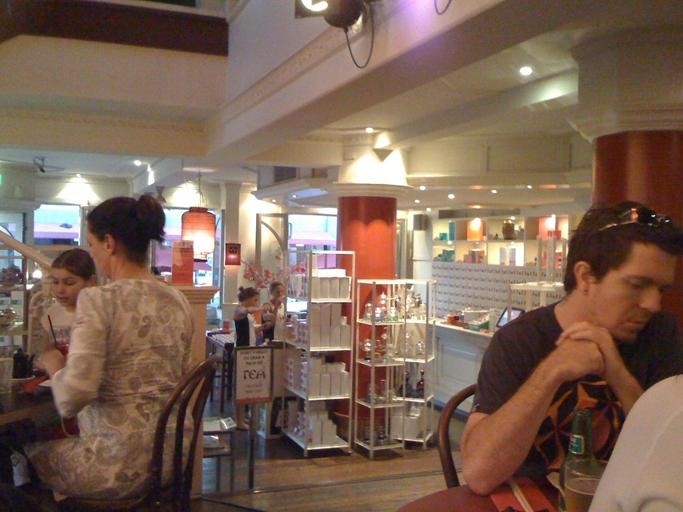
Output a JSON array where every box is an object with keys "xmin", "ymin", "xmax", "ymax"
[
  {"xmin": 339, "ymin": 315, "xmax": 351, "ymax": 347},
  {"xmin": 398, "ymin": 372, "xmax": 412, "ymax": 397},
  {"xmin": 416, "ymin": 369, "xmax": 428, "ymax": 398},
  {"xmin": 559, "ymin": 408, "xmax": 599, "ymax": 512},
  {"xmin": 362, "ymin": 424, "xmax": 385, "ymax": 444},
  {"xmin": 364, "ymin": 290, "xmax": 426, "ymax": 322},
  {"xmin": 366, "ymin": 379, "xmax": 397, "ymax": 403},
  {"xmin": 448, "ymin": 219, "xmax": 456, "ymax": 241},
  {"xmin": 363, "ymin": 328, "xmax": 424, "ymax": 364}
]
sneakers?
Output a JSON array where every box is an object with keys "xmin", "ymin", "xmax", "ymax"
[{"xmin": 236, "ymin": 419, "xmax": 250, "ymax": 431}]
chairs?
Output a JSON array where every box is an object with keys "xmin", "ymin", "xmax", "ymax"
[
  {"xmin": 437, "ymin": 384, "xmax": 476, "ymax": 488},
  {"xmin": 16, "ymin": 355, "xmax": 217, "ymax": 512}
]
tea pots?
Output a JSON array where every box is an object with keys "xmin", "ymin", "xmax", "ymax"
[{"xmin": 13, "ymin": 347, "xmax": 36, "ymax": 378}]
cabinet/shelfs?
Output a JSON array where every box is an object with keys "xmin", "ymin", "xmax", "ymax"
[
  {"xmin": 0, "ymin": 244, "xmax": 27, "ymax": 394},
  {"xmin": 354, "ymin": 278, "xmax": 406, "ymax": 459},
  {"xmin": 390, "ymin": 279, "xmax": 435, "ymax": 450},
  {"xmin": 280, "ymin": 250, "xmax": 356, "ymax": 457},
  {"xmin": 431, "ymin": 202, "xmax": 591, "ymax": 269}
]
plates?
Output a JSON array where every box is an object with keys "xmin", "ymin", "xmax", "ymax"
[{"xmin": 9, "ymin": 375, "xmax": 35, "ymax": 385}]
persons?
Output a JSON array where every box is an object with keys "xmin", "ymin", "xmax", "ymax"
[
  {"xmin": 35, "ymin": 246, "xmax": 97, "ymax": 362},
  {"xmin": 585, "ymin": 375, "xmax": 682, "ymax": 511},
  {"xmin": 457, "ymin": 195, "xmax": 682, "ymax": 502},
  {"xmin": 260, "ymin": 279, "xmax": 289, "ymax": 436},
  {"xmin": 232, "ymin": 287, "xmax": 273, "ymax": 432},
  {"xmin": 7, "ymin": 193, "xmax": 199, "ymax": 512}
]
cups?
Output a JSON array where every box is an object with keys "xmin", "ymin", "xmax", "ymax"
[{"xmin": 48, "ymin": 326, "xmax": 70, "ymax": 356}]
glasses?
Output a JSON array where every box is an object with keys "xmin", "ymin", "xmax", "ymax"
[{"xmin": 600, "ymin": 207, "xmax": 671, "ymax": 231}]
